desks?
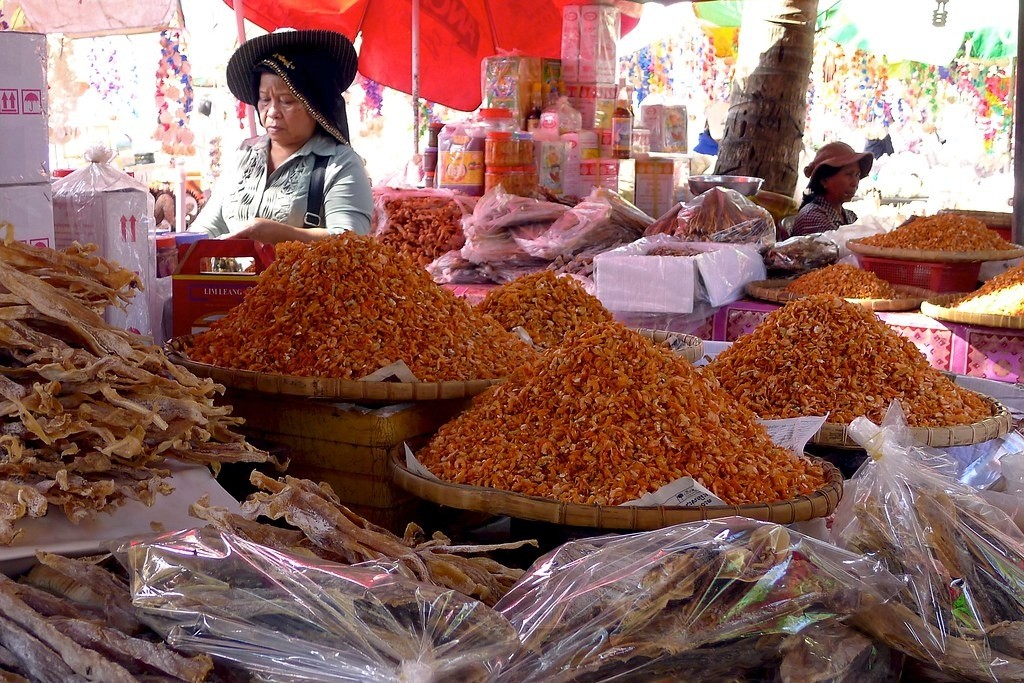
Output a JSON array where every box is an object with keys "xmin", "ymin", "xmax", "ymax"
[
  {"xmin": 712, "ymin": 299, "xmax": 1024, "ymax": 384},
  {"xmin": 0, "ymin": 458, "xmax": 243, "ymax": 578}
]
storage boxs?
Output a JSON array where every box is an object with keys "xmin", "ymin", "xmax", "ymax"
[
  {"xmin": 52, "ymin": 189, "xmax": 173, "ymax": 347},
  {"xmin": 172, "ymin": 239, "xmax": 265, "ymax": 337},
  {"xmin": 0, "ymin": 31, "xmax": 55, "ymax": 251},
  {"xmin": 535, "ymin": 140, "xmax": 564, "ymax": 194},
  {"xmin": 560, "ymin": 127, "xmax": 619, "ymax": 199},
  {"xmin": 595, "ymin": 241, "xmax": 766, "ymax": 313},
  {"xmin": 642, "ymin": 104, "xmax": 688, "ymax": 153},
  {"xmin": 215, "ymin": 388, "xmax": 449, "ymax": 537},
  {"xmin": 486, "ymin": 56, "xmax": 562, "ymax": 130},
  {"xmin": 561, "ymin": 3, "xmax": 621, "ymax": 129},
  {"xmin": 596, "ymin": 99, "xmax": 632, "ymax": 128},
  {"xmin": 635, "ymin": 158, "xmax": 675, "ymax": 220}
]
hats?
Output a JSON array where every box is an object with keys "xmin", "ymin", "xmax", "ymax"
[
  {"xmin": 225, "ymin": 28, "xmax": 358, "ymax": 106},
  {"xmin": 804, "ymin": 140, "xmax": 873, "ymax": 191}
]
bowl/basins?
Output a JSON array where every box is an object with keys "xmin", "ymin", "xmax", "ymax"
[{"xmin": 688, "ymin": 175, "xmax": 765, "ymax": 197}]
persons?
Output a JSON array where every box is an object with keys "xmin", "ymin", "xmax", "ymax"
[
  {"xmin": 184, "ymin": 28, "xmax": 373, "ymax": 272},
  {"xmin": 863, "ymin": 130, "xmax": 893, "ymax": 179},
  {"xmin": 791, "ymin": 141, "xmax": 873, "ymax": 237},
  {"xmin": 904, "ymin": 108, "xmax": 939, "ymax": 153},
  {"xmin": 692, "ymin": 76, "xmax": 734, "ymax": 173}
]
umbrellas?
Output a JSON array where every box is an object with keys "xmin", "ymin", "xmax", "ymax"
[{"xmin": 1, "ymin": 0, "xmax": 1018, "ymax": 184}]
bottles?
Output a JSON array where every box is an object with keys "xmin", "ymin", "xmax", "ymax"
[
  {"xmin": 579, "ymin": 131, "xmax": 598, "ymax": 195},
  {"xmin": 549, "ymin": 87, "xmax": 560, "ymax": 103},
  {"xmin": 599, "ymin": 129, "xmax": 618, "ymax": 194},
  {"xmin": 527, "ymin": 106, "xmax": 540, "ymax": 132},
  {"xmin": 433, "ymin": 124, "xmax": 486, "ymax": 197},
  {"xmin": 612, "ymin": 99, "xmax": 634, "ymax": 158},
  {"xmin": 542, "ymin": 95, "xmax": 582, "ymax": 196}
]
